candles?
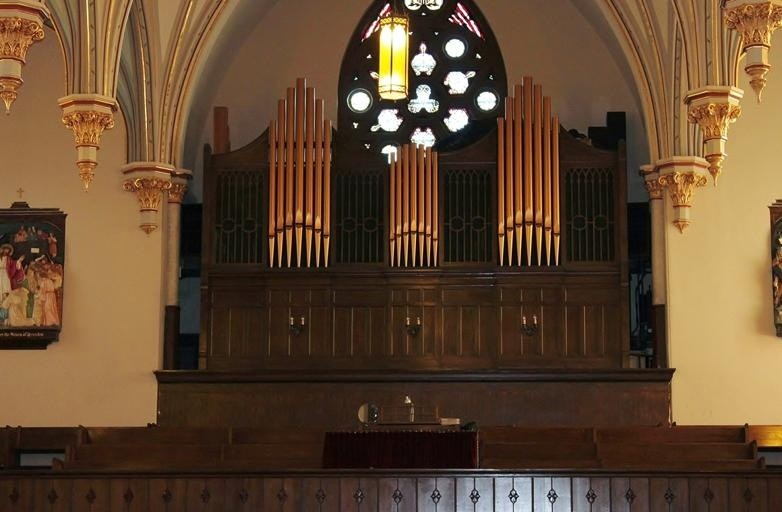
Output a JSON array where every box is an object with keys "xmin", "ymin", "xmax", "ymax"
[
  {"xmin": 406, "ymin": 315, "xmax": 423, "ymax": 336},
  {"xmin": 522, "ymin": 316, "xmax": 539, "ymax": 335},
  {"xmin": 289, "ymin": 312, "xmax": 305, "ymax": 337}
]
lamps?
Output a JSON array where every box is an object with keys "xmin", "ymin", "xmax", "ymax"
[{"xmin": 377, "ymin": 3, "xmax": 414, "ymax": 103}]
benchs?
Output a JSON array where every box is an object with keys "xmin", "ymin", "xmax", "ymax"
[{"xmin": 60, "ymin": 425, "xmax": 782, "ymax": 476}]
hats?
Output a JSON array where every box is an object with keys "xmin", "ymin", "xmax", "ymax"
[{"xmin": 0, "ymin": 243, "xmax": 13, "ymax": 257}]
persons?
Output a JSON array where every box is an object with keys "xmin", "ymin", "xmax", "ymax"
[{"xmin": 1, "ymin": 224, "xmax": 64, "ymax": 333}]
